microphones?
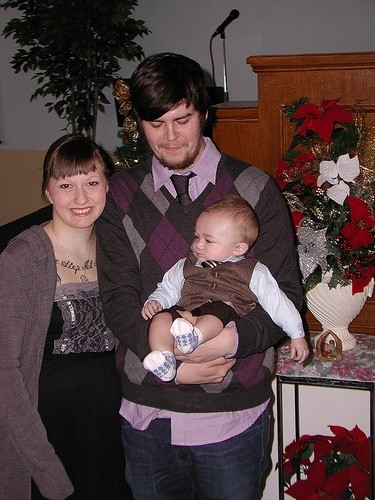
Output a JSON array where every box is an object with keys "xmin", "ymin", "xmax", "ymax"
[{"xmin": 212, "ymin": 9, "xmax": 240, "ymax": 37}]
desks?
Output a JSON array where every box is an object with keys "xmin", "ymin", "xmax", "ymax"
[{"xmin": 277, "ymin": 331, "xmax": 375, "ymax": 500}]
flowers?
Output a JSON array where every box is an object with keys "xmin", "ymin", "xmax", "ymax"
[
  {"xmin": 275, "ymin": 96, "xmax": 375, "ymax": 296},
  {"xmin": 274, "ymin": 424, "xmax": 371, "ymax": 500}
]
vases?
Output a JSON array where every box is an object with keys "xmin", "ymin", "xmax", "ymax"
[{"xmin": 304, "ymin": 268, "xmax": 374, "ymax": 351}]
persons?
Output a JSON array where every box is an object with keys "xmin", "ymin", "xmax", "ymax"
[
  {"xmin": 0, "ymin": 134, "xmax": 136, "ymax": 500},
  {"xmin": 140, "ymin": 194, "xmax": 311, "ymax": 383},
  {"xmin": 322, "ymin": 340, "xmax": 337, "ymax": 359},
  {"xmin": 96, "ymin": 52, "xmax": 304, "ymax": 500}
]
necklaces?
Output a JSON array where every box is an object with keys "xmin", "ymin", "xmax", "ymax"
[{"xmin": 50, "ymin": 220, "xmax": 94, "ymax": 284}]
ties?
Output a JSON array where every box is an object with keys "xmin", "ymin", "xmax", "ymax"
[
  {"xmin": 170, "ymin": 171, "xmax": 197, "ymax": 206},
  {"xmin": 202, "ymin": 259, "xmax": 223, "ymax": 269}
]
